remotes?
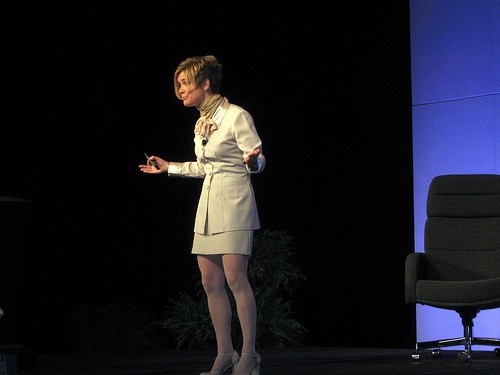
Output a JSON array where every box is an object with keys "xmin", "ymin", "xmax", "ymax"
[{"xmin": 144, "ymin": 153, "xmax": 160, "ymax": 170}]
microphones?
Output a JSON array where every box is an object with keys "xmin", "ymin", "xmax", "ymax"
[
  {"xmin": 188, "ymin": 87, "xmax": 198, "ymax": 94},
  {"xmin": 202, "ymin": 140, "xmax": 207, "ymax": 146}
]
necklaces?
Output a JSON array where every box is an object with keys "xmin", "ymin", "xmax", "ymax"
[{"xmin": 201, "ymin": 131, "xmax": 214, "ymax": 147}]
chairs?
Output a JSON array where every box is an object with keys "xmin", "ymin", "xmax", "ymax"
[{"xmin": 404, "ymin": 173, "xmax": 500, "ymax": 364}]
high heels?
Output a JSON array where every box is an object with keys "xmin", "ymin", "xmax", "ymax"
[
  {"xmin": 231, "ymin": 352, "xmax": 261, "ymax": 375},
  {"xmin": 200, "ymin": 351, "xmax": 241, "ymax": 375}
]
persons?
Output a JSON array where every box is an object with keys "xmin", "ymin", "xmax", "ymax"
[{"xmin": 138, "ymin": 55, "xmax": 266, "ymax": 375}]
